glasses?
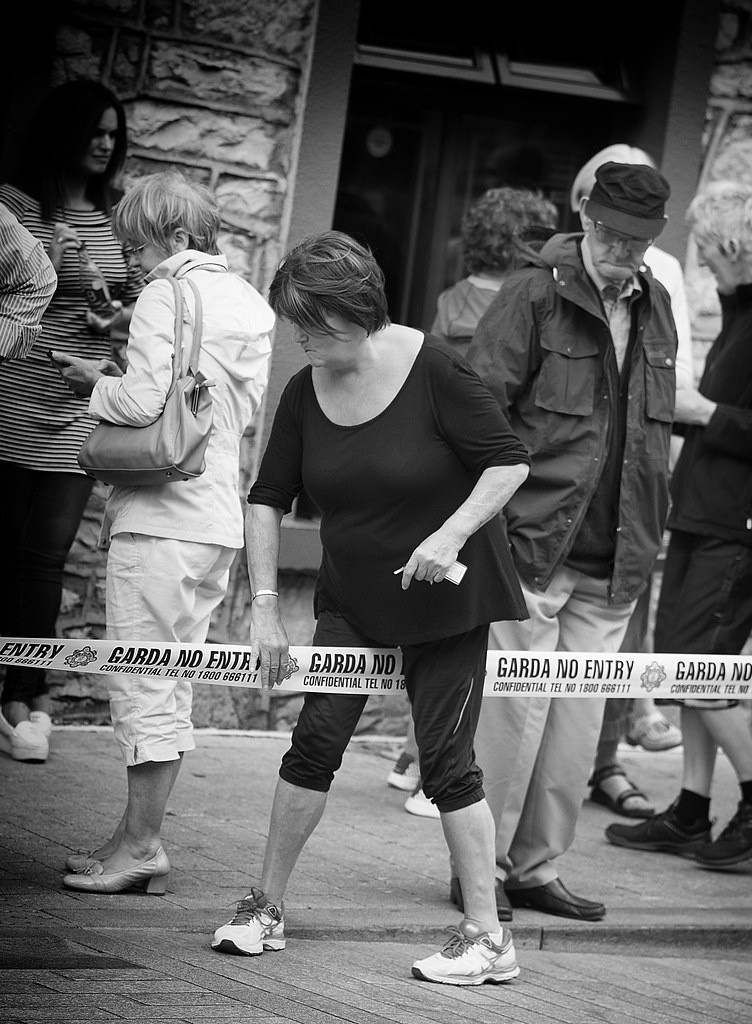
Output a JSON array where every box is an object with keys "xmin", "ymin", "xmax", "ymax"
[
  {"xmin": 588, "ymin": 217, "xmax": 660, "ymax": 252},
  {"xmin": 120, "ymin": 235, "xmax": 164, "ymax": 259}
]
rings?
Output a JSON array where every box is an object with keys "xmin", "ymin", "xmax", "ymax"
[{"xmin": 57, "ymin": 237, "xmax": 65, "ymax": 244}]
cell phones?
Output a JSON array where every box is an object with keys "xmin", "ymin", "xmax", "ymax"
[{"xmin": 45, "ymin": 351, "xmax": 71, "ymax": 368}]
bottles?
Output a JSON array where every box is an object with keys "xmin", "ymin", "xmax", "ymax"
[{"xmin": 74, "ymin": 245, "xmax": 114, "ymax": 318}]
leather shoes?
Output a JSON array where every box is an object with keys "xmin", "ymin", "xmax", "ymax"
[
  {"xmin": 447, "ymin": 872, "xmax": 514, "ymax": 921},
  {"xmin": 503, "ymin": 876, "xmax": 606, "ymax": 922}
]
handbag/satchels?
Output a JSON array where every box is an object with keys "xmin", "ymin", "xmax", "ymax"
[{"xmin": 77, "ymin": 275, "xmax": 215, "ymax": 483}]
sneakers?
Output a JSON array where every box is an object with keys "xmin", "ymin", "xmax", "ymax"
[
  {"xmin": 603, "ymin": 794, "xmax": 719, "ymax": 859},
  {"xmin": 386, "ymin": 752, "xmax": 423, "ymax": 790},
  {"xmin": 28, "ymin": 708, "xmax": 52, "ymax": 737},
  {"xmin": 403, "ymin": 776, "xmax": 442, "ymax": 820},
  {"xmin": 211, "ymin": 885, "xmax": 289, "ymax": 956},
  {"xmin": 696, "ymin": 801, "xmax": 752, "ymax": 871},
  {"xmin": 0, "ymin": 705, "xmax": 49, "ymax": 764},
  {"xmin": 410, "ymin": 919, "xmax": 523, "ymax": 987}
]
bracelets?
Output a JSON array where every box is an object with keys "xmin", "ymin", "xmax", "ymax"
[{"xmin": 251, "ymin": 589, "xmax": 279, "ymax": 600}]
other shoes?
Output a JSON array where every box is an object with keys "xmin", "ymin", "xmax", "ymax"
[{"xmin": 624, "ymin": 711, "xmax": 683, "ymax": 751}]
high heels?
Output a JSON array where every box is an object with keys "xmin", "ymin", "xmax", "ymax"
[
  {"xmin": 66, "ymin": 849, "xmax": 120, "ymax": 873},
  {"xmin": 61, "ymin": 846, "xmax": 172, "ymax": 898}
]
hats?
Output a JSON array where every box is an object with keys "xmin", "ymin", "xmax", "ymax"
[{"xmin": 583, "ymin": 160, "xmax": 674, "ymax": 242}]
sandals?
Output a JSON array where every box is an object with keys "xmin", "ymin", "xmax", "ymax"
[{"xmin": 588, "ymin": 766, "xmax": 656, "ymax": 818}]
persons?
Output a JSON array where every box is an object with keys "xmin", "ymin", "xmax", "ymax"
[
  {"xmin": 1, "ymin": 77, "xmax": 148, "ymax": 762},
  {"xmin": 45, "ymin": 173, "xmax": 281, "ymax": 894},
  {"xmin": 210, "ymin": 227, "xmax": 531, "ymax": 988},
  {"xmin": 0, "ymin": 202, "xmax": 62, "ymax": 362},
  {"xmin": 387, "ymin": 141, "xmax": 752, "ymax": 922}
]
